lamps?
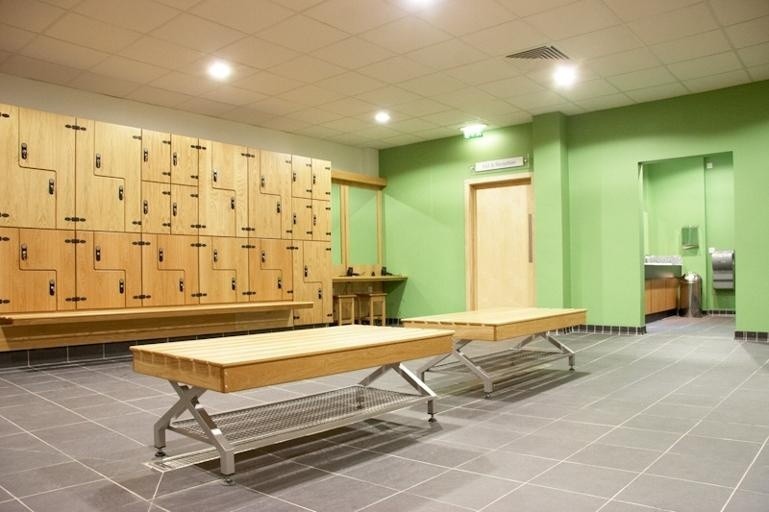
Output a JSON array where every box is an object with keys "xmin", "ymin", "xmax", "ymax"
[{"xmin": 458, "ymin": 124, "xmax": 488, "ymax": 138}]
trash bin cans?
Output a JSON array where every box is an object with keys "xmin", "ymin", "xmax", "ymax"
[{"xmin": 679, "ymin": 271, "xmax": 704, "ymax": 318}]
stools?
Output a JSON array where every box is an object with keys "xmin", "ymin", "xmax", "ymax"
[
  {"xmin": 334, "ymin": 294, "xmax": 357, "ymax": 325},
  {"xmin": 357, "ymin": 292, "xmax": 388, "ymax": 326}
]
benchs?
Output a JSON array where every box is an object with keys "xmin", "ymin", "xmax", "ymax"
[{"xmin": 0, "ymin": 298, "xmax": 311, "ymax": 363}]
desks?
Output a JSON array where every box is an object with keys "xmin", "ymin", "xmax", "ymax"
[
  {"xmin": 332, "ymin": 275, "xmax": 407, "ymax": 283},
  {"xmin": 129, "ymin": 325, "xmax": 453, "ymax": 476},
  {"xmin": 401, "ymin": 305, "xmax": 587, "ymax": 396}
]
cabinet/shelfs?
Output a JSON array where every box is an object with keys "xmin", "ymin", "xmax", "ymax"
[
  {"xmin": 0, "ymin": 103, "xmax": 335, "ymax": 325},
  {"xmin": 645, "ymin": 278, "xmax": 681, "ymax": 316}
]
